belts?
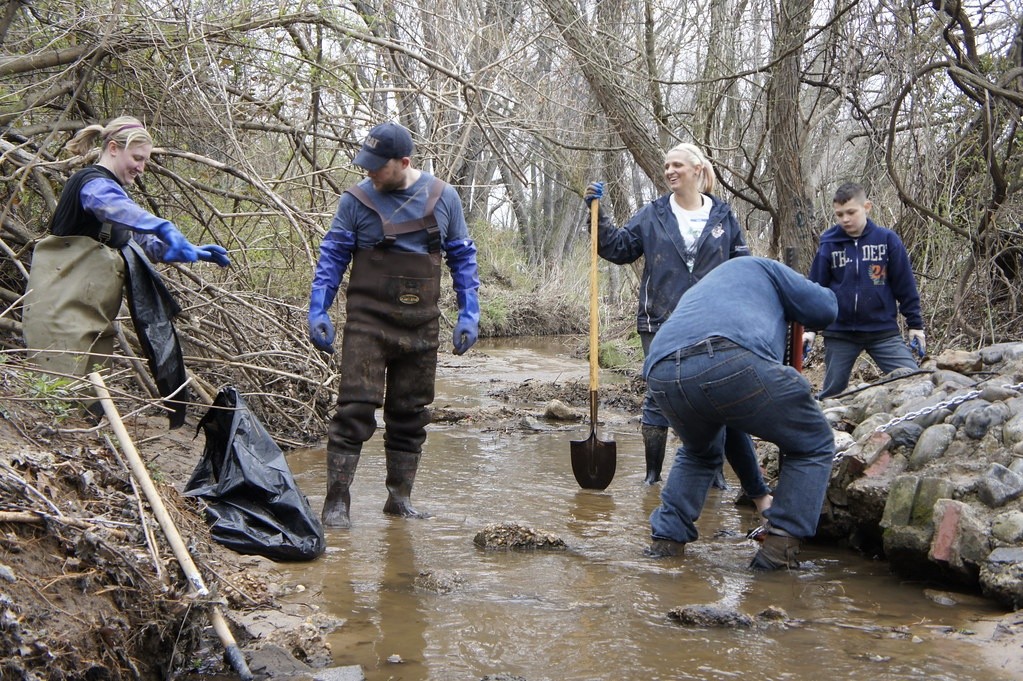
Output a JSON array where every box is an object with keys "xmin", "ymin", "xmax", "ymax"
[{"xmin": 663, "ymin": 340, "xmax": 740, "ymax": 360}]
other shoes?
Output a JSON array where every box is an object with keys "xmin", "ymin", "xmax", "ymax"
[
  {"xmin": 646, "ymin": 534, "xmax": 684, "ymax": 557},
  {"xmin": 747, "ymin": 532, "xmax": 817, "ymax": 573}
]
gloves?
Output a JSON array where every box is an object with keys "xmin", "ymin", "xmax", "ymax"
[
  {"xmin": 443, "ymin": 238, "xmax": 479, "ymax": 356},
  {"xmin": 909, "ymin": 329, "xmax": 926, "ymax": 358},
  {"xmin": 801, "ymin": 331, "xmax": 816, "ymax": 360},
  {"xmin": 134, "ymin": 229, "xmax": 231, "ymax": 267},
  {"xmin": 583, "ymin": 181, "xmax": 604, "ymax": 212},
  {"xmin": 308, "ymin": 229, "xmax": 354, "ymax": 354},
  {"xmin": 81, "ymin": 178, "xmax": 211, "ymax": 261}
]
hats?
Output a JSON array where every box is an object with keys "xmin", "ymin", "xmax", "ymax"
[{"xmin": 352, "ymin": 123, "xmax": 413, "ymax": 170}]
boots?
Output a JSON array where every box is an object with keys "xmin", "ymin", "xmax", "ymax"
[
  {"xmin": 642, "ymin": 424, "xmax": 668, "ymax": 484},
  {"xmin": 711, "ymin": 469, "xmax": 727, "ymax": 490},
  {"xmin": 321, "ymin": 450, "xmax": 360, "ymax": 528},
  {"xmin": 383, "ymin": 450, "xmax": 431, "ymax": 518}
]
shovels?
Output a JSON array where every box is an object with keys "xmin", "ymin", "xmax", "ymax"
[{"xmin": 570, "ymin": 198, "xmax": 620, "ymax": 491}]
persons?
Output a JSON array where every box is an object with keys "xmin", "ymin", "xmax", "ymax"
[
  {"xmin": 642, "ymin": 256, "xmax": 839, "ymax": 570},
  {"xmin": 584, "ymin": 143, "xmax": 751, "ymax": 491},
  {"xmin": 308, "ymin": 121, "xmax": 480, "ymax": 528},
  {"xmin": 22, "ymin": 115, "xmax": 231, "ymax": 427},
  {"xmin": 801, "ymin": 183, "xmax": 925, "ymax": 401}
]
